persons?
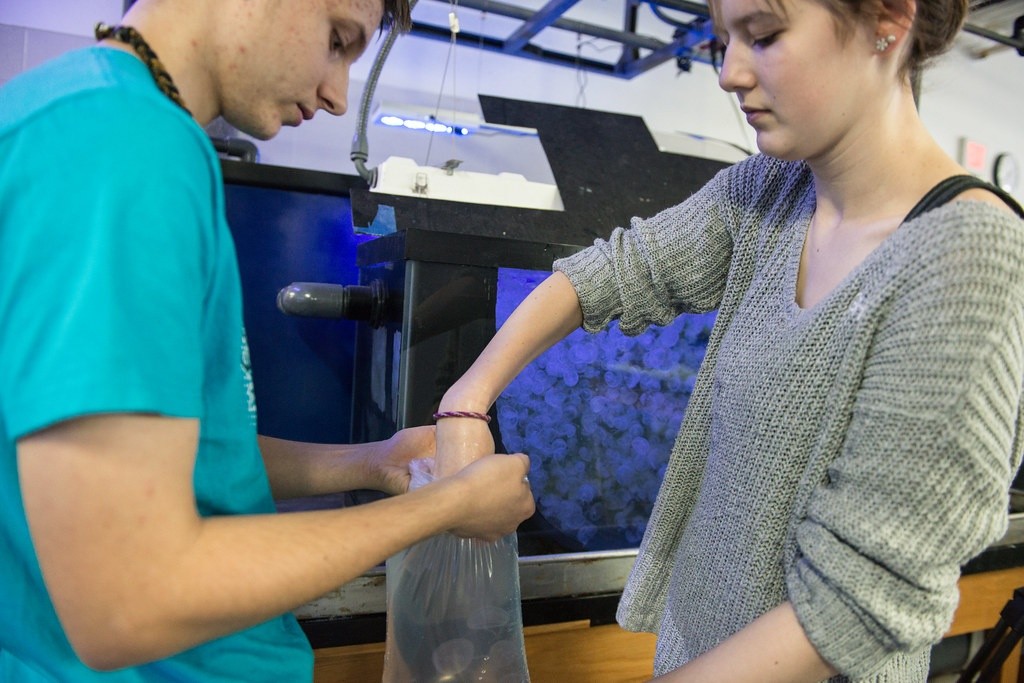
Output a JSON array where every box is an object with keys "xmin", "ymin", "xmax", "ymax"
[
  {"xmin": 429, "ymin": 2, "xmax": 1024, "ymax": 683},
  {"xmin": 0, "ymin": 0, "xmax": 536, "ymax": 682}
]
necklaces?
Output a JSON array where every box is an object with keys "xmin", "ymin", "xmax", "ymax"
[{"xmin": 94, "ymin": 19, "xmax": 193, "ymax": 117}]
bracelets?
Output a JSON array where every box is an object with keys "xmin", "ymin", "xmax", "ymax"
[{"xmin": 431, "ymin": 411, "xmax": 492, "ymax": 423}]
